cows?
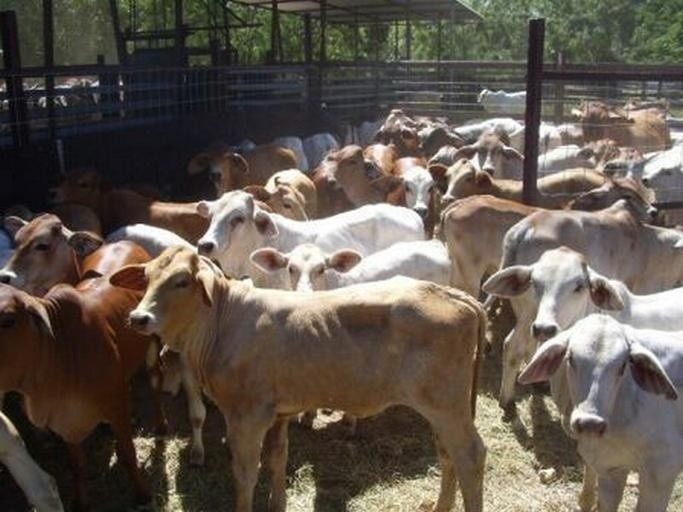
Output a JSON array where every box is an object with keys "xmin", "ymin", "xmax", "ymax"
[{"xmin": 1, "ymin": 79, "xmax": 682, "ymax": 512}]
